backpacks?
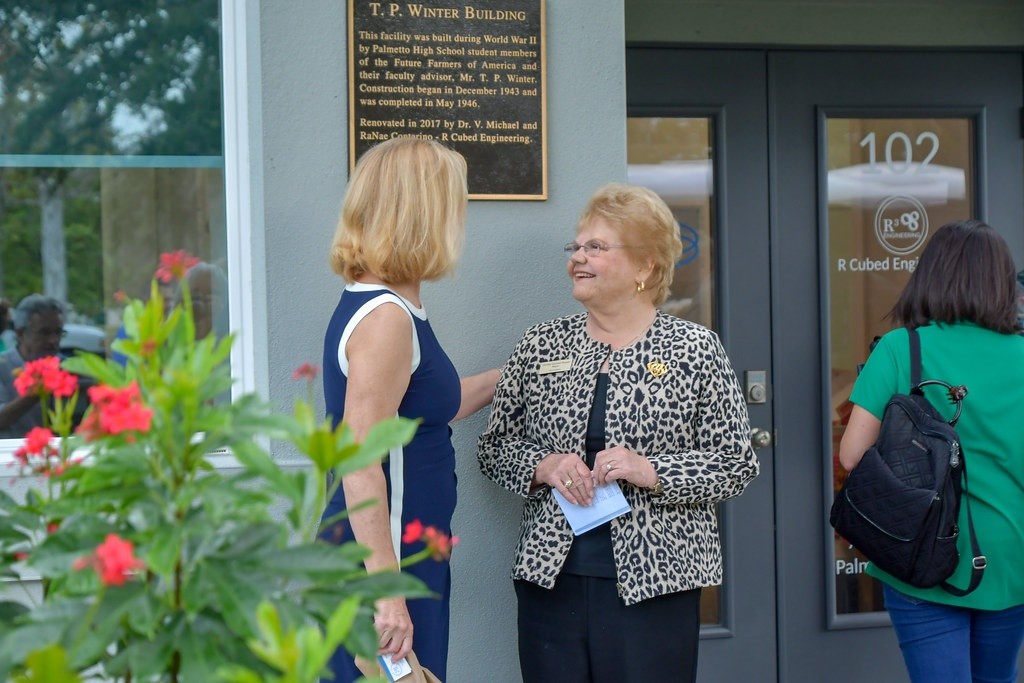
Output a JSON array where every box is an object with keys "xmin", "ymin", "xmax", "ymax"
[{"xmin": 827, "ymin": 326, "xmax": 987, "ymax": 597}]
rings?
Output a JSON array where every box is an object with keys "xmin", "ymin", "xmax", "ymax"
[
  {"xmin": 607, "ymin": 464, "xmax": 612, "ymax": 471},
  {"xmin": 565, "ymin": 480, "xmax": 572, "ymax": 488}
]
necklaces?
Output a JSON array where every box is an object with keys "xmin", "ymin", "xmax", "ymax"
[{"xmin": 620, "ymin": 323, "xmax": 651, "ymax": 347}]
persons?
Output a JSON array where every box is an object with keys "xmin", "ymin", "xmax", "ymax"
[
  {"xmin": 315, "ymin": 138, "xmax": 499, "ymax": 683},
  {"xmin": 476, "ymin": 184, "xmax": 760, "ymax": 683},
  {"xmin": 0, "ymin": 261, "xmax": 231, "ymax": 438},
  {"xmin": 839, "ymin": 221, "xmax": 1024, "ymax": 683}
]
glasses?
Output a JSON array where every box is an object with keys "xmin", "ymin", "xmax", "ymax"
[
  {"xmin": 24, "ymin": 328, "xmax": 68, "ymax": 337},
  {"xmin": 562, "ymin": 238, "xmax": 627, "ymax": 258}
]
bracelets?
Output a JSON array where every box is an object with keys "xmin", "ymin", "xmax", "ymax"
[{"xmin": 645, "ymin": 480, "xmax": 664, "ymax": 493}]
